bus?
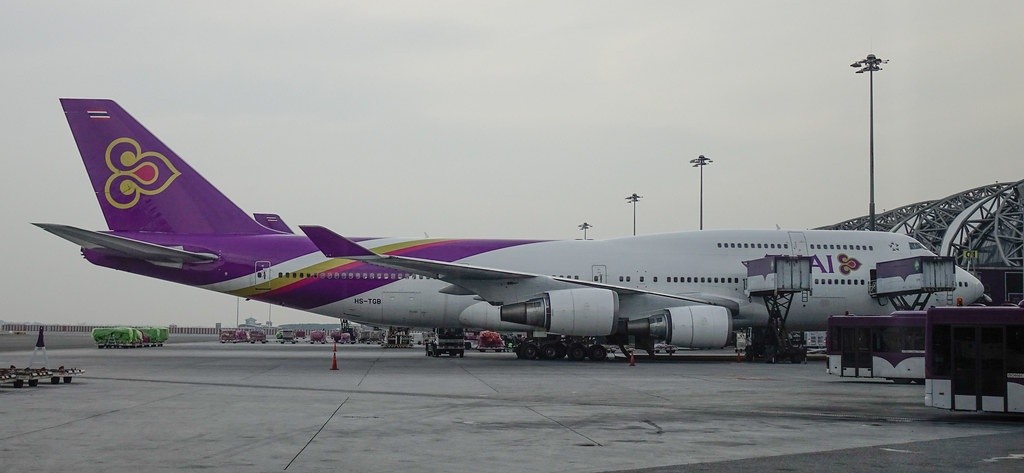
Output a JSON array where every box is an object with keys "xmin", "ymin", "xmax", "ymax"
[
  {"xmin": 826, "ymin": 308, "xmax": 932, "ymax": 385},
  {"xmin": 923, "ymin": 303, "xmax": 1024, "ymax": 420}
]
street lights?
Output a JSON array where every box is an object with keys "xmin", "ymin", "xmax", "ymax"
[
  {"xmin": 579, "ymin": 221, "xmax": 593, "ymax": 241},
  {"xmin": 626, "ymin": 190, "xmax": 644, "ymax": 237},
  {"xmin": 849, "ymin": 52, "xmax": 891, "ymax": 233},
  {"xmin": 688, "ymin": 152, "xmax": 717, "ymax": 231}
]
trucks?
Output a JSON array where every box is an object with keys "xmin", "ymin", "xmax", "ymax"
[{"xmin": 425, "ymin": 327, "xmax": 466, "ymax": 360}]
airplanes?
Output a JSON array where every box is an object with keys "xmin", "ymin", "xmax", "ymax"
[{"xmin": 27, "ymin": 93, "xmax": 988, "ymax": 366}]
